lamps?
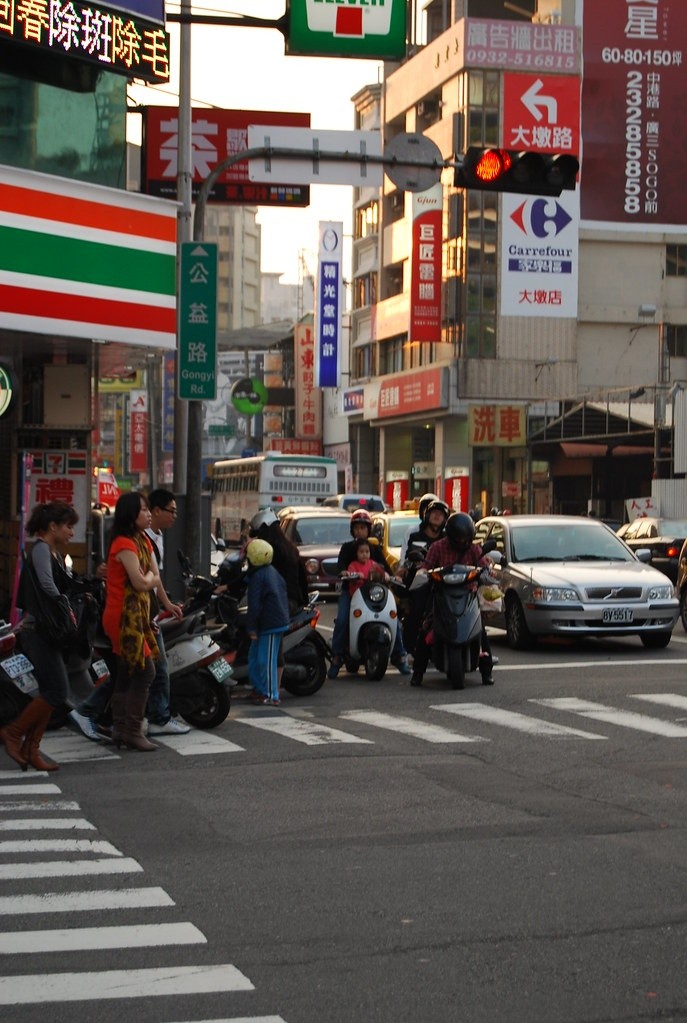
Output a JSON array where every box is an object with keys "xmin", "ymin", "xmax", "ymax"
[{"xmin": 637, "ymin": 304, "xmax": 657, "ymax": 324}]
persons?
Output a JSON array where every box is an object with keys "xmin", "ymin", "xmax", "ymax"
[
  {"xmin": 0, "ymin": 502, "xmax": 79, "ymax": 772},
  {"xmin": 348, "ymin": 539, "xmax": 390, "ymax": 598},
  {"xmin": 213, "ymin": 509, "xmax": 309, "ymax": 688},
  {"xmin": 403, "ymin": 493, "xmax": 498, "ymax": 688},
  {"xmin": 245, "ymin": 539, "xmax": 290, "ymax": 706},
  {"xmin": 589, "ymin": 511, "xmax": 596, "ymax": 520},
  {"xmin": 327, "ymin": 510, "xmax": 411, "ymax": 679},
  {"xmin": 469, "ymin": 507, "xmax": 512, "ymax": 524},
  {"xmin": 69, "ymin": 489, "xmax": 190, "ymax": 751}
]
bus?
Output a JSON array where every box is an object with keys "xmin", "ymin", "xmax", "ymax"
[{"xmin": 203, "ymin": 452, "xmax": 339, "ymax": 550}]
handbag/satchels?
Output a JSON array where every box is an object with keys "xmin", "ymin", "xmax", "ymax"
[{"xmin": 35, "ymin": 601, "xmax": 83, "ymax": 651}]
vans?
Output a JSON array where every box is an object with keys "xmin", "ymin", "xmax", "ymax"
[{"xmin": 320, "ymin": 492, "xmax": 389, "ymax": 514}]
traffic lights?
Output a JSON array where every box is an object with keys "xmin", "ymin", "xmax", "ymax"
[{"xmin": 461, "ymin": 147, "xmax": 579, "ymax": 192}]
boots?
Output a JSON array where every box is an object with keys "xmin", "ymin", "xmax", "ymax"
[
  {"xmin": 0, "ymin": 695, "xmax": 52, "ymax": 765},
  {"xmin": 112, "ymin": 690, "xmax": 126, "ymax": 751},
  {"xmin": 121, "ymin": 688, "xmax": 159, "ymax": 751},
  {"xmin": 19, "ymin": 705, "xmax": 60, "ymax": 771}
]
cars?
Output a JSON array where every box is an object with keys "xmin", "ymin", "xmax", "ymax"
[
  {"xmin": 369, "ymin": 508, "xmax": 454, "ymax": 577},
  {"xmin": 614, "ymin": 514, "xmax": 687, "ymax": 633},
  {"xmin": 210, "ymin": 531, "xmax": 228, "ymax": 577},
  {"xmin": 275, "ymin": 504, "xmax": 358, "ymax": 591},
  {"xmin": 472, "ymin": 512, "xmax": 680, "ymax": 653}
]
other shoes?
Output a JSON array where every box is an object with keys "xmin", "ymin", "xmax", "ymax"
[
  {"xmin": 391, "ymin": 658, "xmax": 411, "ymax": 674},
  {"xmin": 482, "ymin": 675, "xmax": 494, "ymax": 685},
  {"xmin": 328, "ymin": 660, "xmax": 343, "ymax": 678},
  {"xmin": 246, "ymin": 690, "xmax": 280, "ymax": 705},
  {"xmin": 411, "ymin": 669, "xmax": 423, "ymax": 686}
]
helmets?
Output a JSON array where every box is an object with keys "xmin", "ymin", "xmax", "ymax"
[
  {"xmin": 445, "ymin": 514, "xmax": 474, "ymax": 552},
  {"xmin": 424, "ymin": 500, "xmax": 449, "ymax": 527},
  {"xmin": 350, "ymin": 509, "xmax": 372, "ymax": 537},
  {"xmin": 246, "ymin": 539, "xmax": 274, "ymax": 567},
  {"xmin": 249, "ymin": 510, "xmax": 280, "ymax": 532},
  {"xmin": 419, "ymin": 493, "xmax": 440, "ymax": 519}
]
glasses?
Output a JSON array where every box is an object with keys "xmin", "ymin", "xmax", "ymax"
[{"xmin": 159, "ymin": 507, "xmax": 177, "ymax": 515}]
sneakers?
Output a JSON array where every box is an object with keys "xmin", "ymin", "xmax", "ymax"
[
  {"xmin": 147, "ymin": 718, "xmax": 191, "ymax": 736},
  {"xmin": 67, "ymin": 709, "xmax": 101, "ymax": 741}
]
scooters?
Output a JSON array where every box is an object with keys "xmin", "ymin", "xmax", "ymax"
[
  {"xmin": 329, "ymin": 573, "xmax": 407, "ymax": 683},
  {"xmin": 0, "ymin": 552, "xmax": 238, "ymax": 730},
  {"xmin": 403, "ymin": 539, "xmax": 497, "ymax": 692},
  {"xmin": 173, "ymin": 549, "xmax": 338, "ymax": 698}
]
